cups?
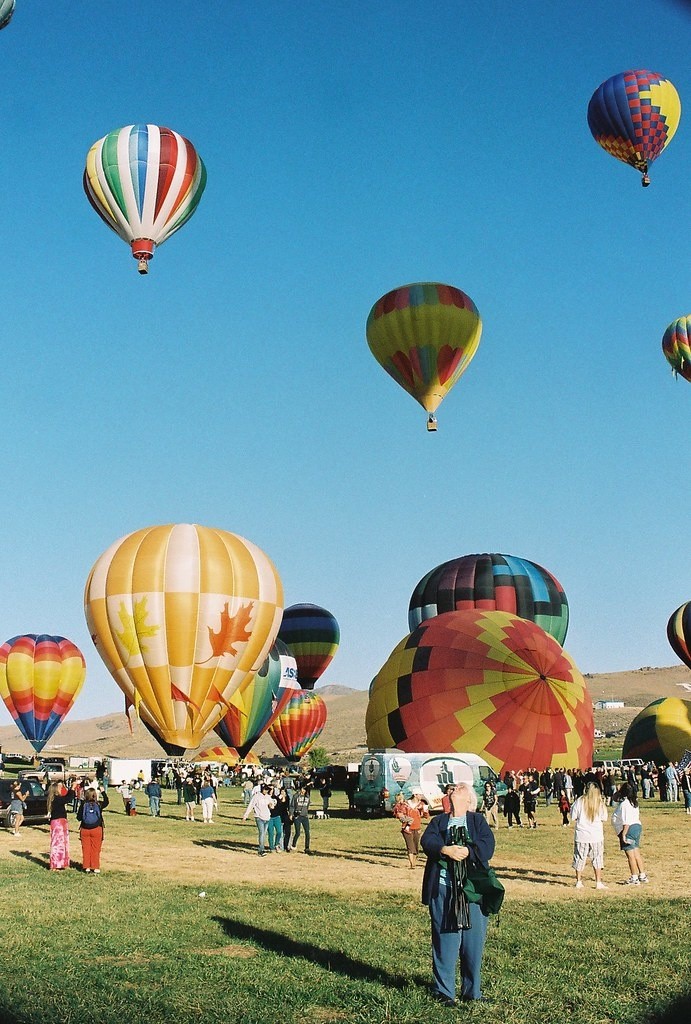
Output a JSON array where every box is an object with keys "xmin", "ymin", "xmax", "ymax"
[{"xmin": 423, "ymin": 805, "xmax": 428, "ymax": 812}]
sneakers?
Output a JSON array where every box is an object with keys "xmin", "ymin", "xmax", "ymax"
[
  {"xmin": 562, "ymin": 821, "xmax": 567, "ymax": 827},
  {"xmin": 12, "ymin": 830, "xmax": 22, "ymax": 837},
  {"xmin": 624, "ymin": 877, "xmax": 640, "ymax": 884},
  {"xmin": 576, "ymin": 881, "xmax": 585, "ymax": 888},
  {"xmin": 258, "ymin": 851, "xmax": 267, "ymax": 857},
  {"xmin": 637, "ymin": 875, "xmax": 649, "ymax": 883},
  {"xmin": 565, "ymin": 820, "xmax": 570, "ymax": 826},
  {"xmin": 595, "ymin": 883, "xmax": 606, "ymax": 889}
]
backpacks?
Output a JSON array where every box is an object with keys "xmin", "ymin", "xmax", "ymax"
[{"xmin": 83, "ymin": 802, "xmax": 101, "ymax": 828}]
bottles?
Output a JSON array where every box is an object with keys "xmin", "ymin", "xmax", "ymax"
[{"xmin": 626, "ymin": 838, "xmax": 635, "ymax": 844}]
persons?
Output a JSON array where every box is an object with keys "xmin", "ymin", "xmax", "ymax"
[
  {"xmin": 241, "ymin": 764, "xmax": 335, "ymax": 856},
  {"xmin": 392, "ymin": 788, "xmax": 429, "ymax": 869},
  {"xmin": 115, "ymin": 766, "xmax": 166, "ymax": 817},
  {"xmin": 0, "ymin": 759, "xmax": 110, "ymax": 836},
  {"xmin": 165, "ymin": 763, "xmax": 242, "ymax": 823},
  {"xmin": 344, "ymin": 774, "xmax": 354, "ymax": 804},
  {"xmin": 611, "ymin": 783, "xmax": 649, "ymax": 885},
  {"xmin": 47, "ymin": 782, "xmax": 77, "ymax": 872},
  {"xmin": 76, "ymin": 786, "xmax": 109, "ymax": 873},
  {"xmin": 320, "ymin": 784, "xmax": 331, "ymax": 810},
  {"xmin": 504, "ymin": 761, "xmax": 691, "ymax": 830},
  {"xmin": 479, "ymin": 781, "xmax": 499, "ymax": 830},
  {"xmin": 420, "ymin": 783, "xmax": 495, "ymax": 1007},
  {"xmin": 570, "ymin": 781, "xmax": 608, "ymax": 889},
  {"xmin": 442, "ymin": 784, "xmax": 453, "ymax": 813}
]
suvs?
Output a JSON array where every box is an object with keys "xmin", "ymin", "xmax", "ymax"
[{"xmin": 0, "ymin": 778, "xmax": 53, "ymax": 828}]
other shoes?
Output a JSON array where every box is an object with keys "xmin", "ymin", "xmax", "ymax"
[
  {"xmin": 408, "ymin": 861, "xmax": 416, "ymax": 869},
  {"xmin": 487, "ymin": 821, "xmax": 538, "ymax": 828},
  {"xmin": 86, "ymin": 868, "xmax": 90, "ymax": 872},
  {"xmin": 94, "ymin": 869, "xmax": 100, "ymax": 873},
  {"xmin": 270, "ymin": 846, "xmax": 314, "ymax": 854}
]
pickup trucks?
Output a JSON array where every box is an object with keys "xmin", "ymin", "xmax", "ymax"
[{"xmin": 18, "ymin": 763, "xmax": 97, "ymax": 785}]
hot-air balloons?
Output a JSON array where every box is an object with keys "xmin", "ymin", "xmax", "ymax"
[
  {"xmin": 267, "ymin": 689, "xmax": 327, "ymax": 765},
  {"xmin": 82, "ymin": 124, "xmax": 205, "ymax": 274},
  {"xmin": 83, "ymin": 523, "xmax": 284, "ymax": 767},
  {"xmin": 212, "ymin": 638, "xmax": 298, "ymax": 765},
  {"xmin": 586, "ymin": 70, "xmax": 681, "ymax": 187},
  {"xmin": 662, "ymin": 314, "xmax": 691, "ymax": 384},
  {"xmin": 409, "ymin": 553, "xmax": 569, "ymax": 648},
  {"xmin": 190, "ymin": 746, "xmax": 264, "ymax": 769},
  {"xmin": 364, "ymin": 609, "xmax": 595, "ymax": 781},
  {"xmin": 0, "ymin": 634, "xmax": 87, "ymax": 772},
  {"xmin": 667, "ymin": 600, "xmax": 691, "ymax": 671},
  {"xmin": 277, "ymin": 602, "xmax": 341, "ymax": 690},
  {"xmin": 366, "ymin": 282, "xmax": 483, "ymax": 433},
  {"xmin": 621, "ymin": 696, "xmax": 691, "ymax": 767}
]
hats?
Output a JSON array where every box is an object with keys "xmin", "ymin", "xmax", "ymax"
[
  {"xmin": 80, "ymin": 777, "xmax": 91, "ymax": 787},
  {"xmin": 412, "ymin": 788, "xmax": 424, "ymax": 794}
]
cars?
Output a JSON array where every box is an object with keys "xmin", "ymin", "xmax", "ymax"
[
  {"xmin": 594, "ymin": 728, "xmax": 621, "ymax": 739},
  {"xmin": 233, "ymin": 764, "xmax": 264, "ymax": 778}
]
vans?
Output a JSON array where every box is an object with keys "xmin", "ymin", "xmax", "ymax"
[
  {"xmin": 617, "ymin": 758, "xmax": 644, "ymax": 767},
  {"xmin": 592, "ymin": 760, "xmax": 621, "ymax": 774},
  {"xmin": 352, "ymin": 747, "xmax": 509, "ymax": 819}
]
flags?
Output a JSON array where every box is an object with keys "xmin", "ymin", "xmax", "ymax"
[{"xmin": 675, "ymin": 749, "xmax": 691, "ymax": 776}]
